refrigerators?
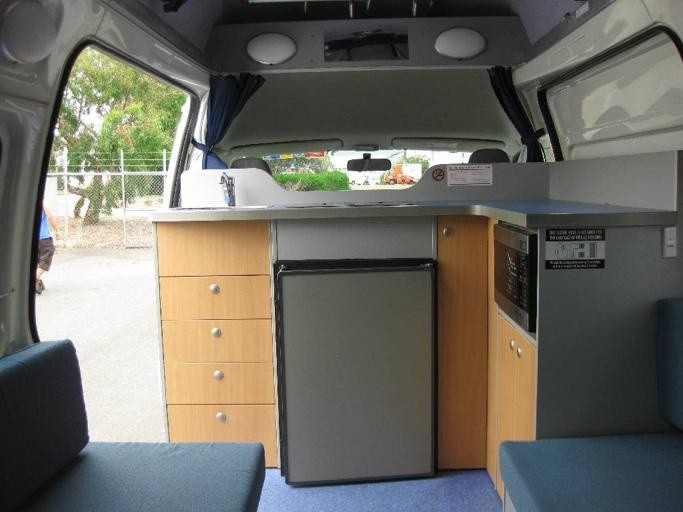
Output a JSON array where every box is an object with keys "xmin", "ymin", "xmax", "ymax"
[{"xmin": 272, "ymin": 262, "xmax": 438, "ymax": 486}]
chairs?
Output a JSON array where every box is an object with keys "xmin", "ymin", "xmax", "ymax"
[
  {"xmin": 468, "ymin": 149, "xmax": 510, "ymax": 163},
  {"xmin": 230, "ymin": 157, "xmax": 273, "ymax": 176},
  {"xmin": 499, "ymin": 299, "xmax": 683, "ymax": 510},
  {"xmin": 0, "ymin": 340, "xmax": 264, "ymax": 512}
]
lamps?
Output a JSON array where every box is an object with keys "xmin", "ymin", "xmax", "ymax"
[
  {"xmin": 3, "ymin": 1, "xmax": 56, "ymax": 67},
  {"xmin": 245, "ymin": 32, "xmax": 296, "ymax": 65},
  {"xmin": 434, "ymin": 26, "xmax": 487, "ymax": 58}
]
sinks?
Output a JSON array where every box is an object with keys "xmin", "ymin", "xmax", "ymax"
[{"xmin": 172, "ymin": 206, "xmax": 273, "ymax": 210}]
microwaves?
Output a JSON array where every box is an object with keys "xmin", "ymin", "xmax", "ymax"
[{"xmin": 493, "ymin": 222, "xmax": 537, "ymax": 334}]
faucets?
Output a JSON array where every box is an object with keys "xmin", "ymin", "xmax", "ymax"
[{"xmin": 219, "ymin": 171, "xmax": 236, "ymax": 207}]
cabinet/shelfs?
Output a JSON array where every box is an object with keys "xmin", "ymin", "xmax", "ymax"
[
  {"xmin": 496, "ymin": 315, "xmax": 535, "ymax": 500},
  {"xmin": 438, "ymin": 215, "xmax": 487, "ymax": 471},
  {"xmin": 156, "ymin": 220, "xmax": 278, "ymax": 466}
]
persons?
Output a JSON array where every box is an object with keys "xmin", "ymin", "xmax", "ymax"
[{"xmin": 35, "ymin": 207, "xmax": 55, "ymax": 294}]
